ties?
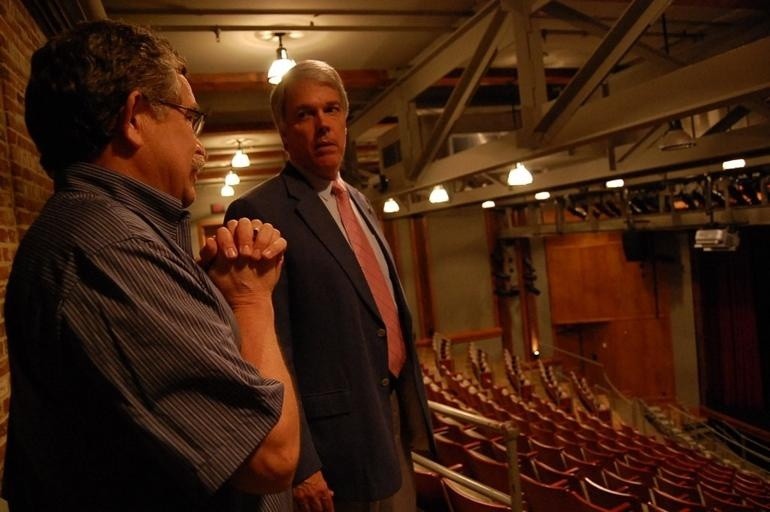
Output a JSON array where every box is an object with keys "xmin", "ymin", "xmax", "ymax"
[{"xmin": 330, "ymin": 181, "xmax": 407, "ymax": 380}]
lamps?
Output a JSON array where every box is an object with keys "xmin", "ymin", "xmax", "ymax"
[
  {"xmin": 267, "ymin": 31, "xmax": 298, "ymax": 86},
  {"xmin": 659, "ymin": 120, "xmax": 695, "ymax": 153}
]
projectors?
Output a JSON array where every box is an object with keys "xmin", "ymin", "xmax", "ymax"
[{"xmin": 695, "ymin": 229, "xmax": 740, "ymax": 252}]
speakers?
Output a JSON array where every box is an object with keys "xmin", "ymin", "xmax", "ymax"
[{"xmin": 623, "ymin": 230, "xmax": 660, "ymax": 261}]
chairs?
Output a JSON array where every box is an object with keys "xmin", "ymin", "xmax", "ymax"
[{"xmin": 404, "ymin": 331, "xmax": 770, "ymax": 510}]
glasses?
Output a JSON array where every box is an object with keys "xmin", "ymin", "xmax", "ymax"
[{"xmin": 159, "ymin": 99, "xmax": 208, "ymax": 139}]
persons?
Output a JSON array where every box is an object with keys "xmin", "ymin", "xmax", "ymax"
[
  {"xmin": 0, "ymin": 19, "xmax": 301, "ymax": 512},
  {"xmin": 223, "ymin": 60, "xmax": 438, "ymax": 512}
]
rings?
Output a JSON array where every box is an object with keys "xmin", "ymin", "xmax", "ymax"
[{"xmin": 253, "ymin": 227, "xmax": 259, "ymax": 232}]
